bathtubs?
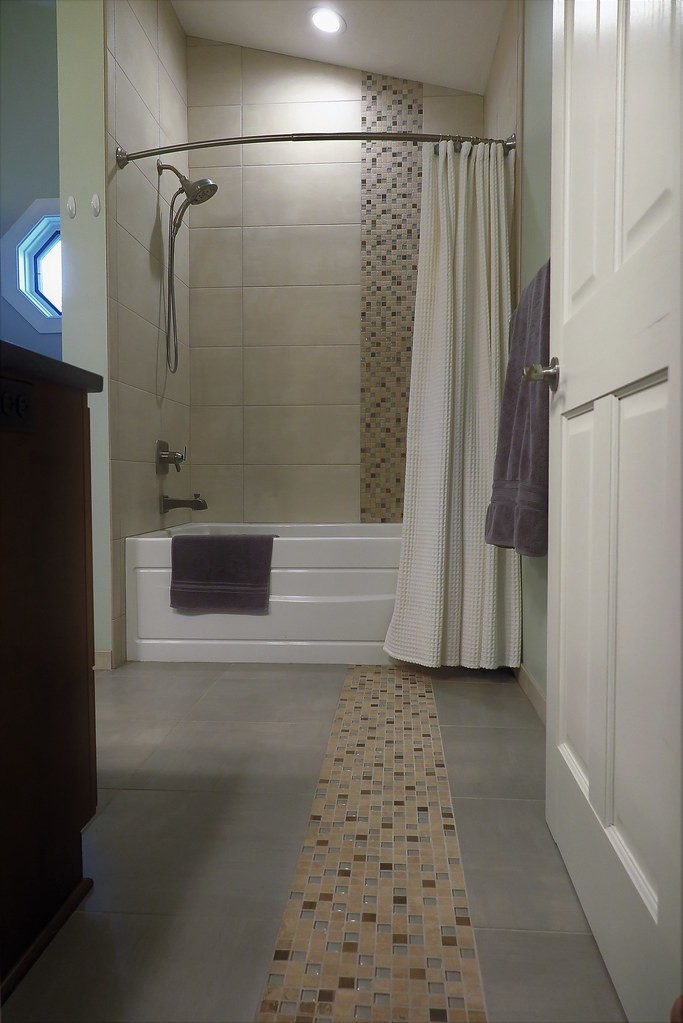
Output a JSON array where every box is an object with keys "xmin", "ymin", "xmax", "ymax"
[{"xmin": 125, "ymin": 521, "xmax": 416, "ymax": 665}]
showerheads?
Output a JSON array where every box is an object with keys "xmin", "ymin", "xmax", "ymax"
[{"xmin": 157, "ymin": 158, "xmax": 219, "ymax": 206}]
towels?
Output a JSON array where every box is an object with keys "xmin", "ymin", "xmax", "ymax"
[
  {"xmin": 483, "ymin": 257, "xmax": 551, "ymax": 558},
  {"xmin": 169, "ymin": 533, "xmax": 281, "ymax": 615}
]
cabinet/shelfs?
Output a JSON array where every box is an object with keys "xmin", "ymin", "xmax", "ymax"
[{"xmin": 0, "ymin": 338, "xmax": 105, "ymax": 1007}]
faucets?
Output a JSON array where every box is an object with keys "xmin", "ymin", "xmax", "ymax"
[{"xmin": 159, "ymin": 495, "xmax": 209, "ymax": 514}]
